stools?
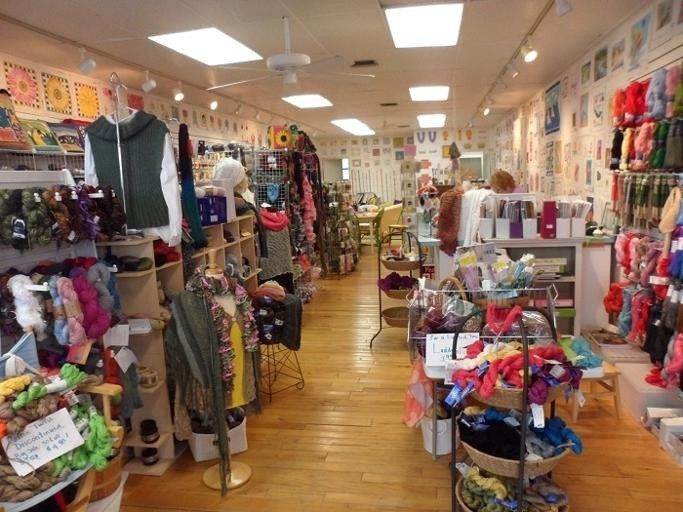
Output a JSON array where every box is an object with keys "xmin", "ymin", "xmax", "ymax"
[
  {"xmin": 389, "ymin": 224, "xmax": 408, "ymax": 246},
  {"xmin": 563, "ymin": 359, "xmax": 623, "ymax": 424}
]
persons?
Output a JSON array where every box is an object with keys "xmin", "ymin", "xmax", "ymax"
[
  {"xmin": 487, "ymin": 170, "xmax": 527, "ymax": 193},
  {"xmin": 211, "ymin": 156, "xmax": 250, "ymax": 214},
  {"xmin": 162, "ymin": 264, "xmax": 258, "ymax": 428},
  {"xmin": 256, "ymin": 201, "xmax": 296, "ymax": 296},
  {"xmin": 83, "ymin": 73, "xmax": 183, "ymax": 248}
]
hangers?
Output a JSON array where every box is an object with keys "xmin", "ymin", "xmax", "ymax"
[{"xmin": 108, "ymin": 84, "xmax": 134, "ymax": 115}]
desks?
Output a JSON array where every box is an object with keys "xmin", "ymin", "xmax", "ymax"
[{"xmin": 417, "ymin": 364, "xmax": 603, "ymax": 459}]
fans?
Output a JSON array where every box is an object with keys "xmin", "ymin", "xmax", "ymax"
[{"xmin": 205, "ymin": 16, "xmax": 377, "ymax": 92}]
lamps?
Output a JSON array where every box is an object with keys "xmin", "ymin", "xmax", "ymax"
[
  {"xmin": 342, "ymin": 158, "xmax": 350, "ymax": 181},
  {"xmin": 77, "ymin": 46, "xmax": 328, "ymax": 138},
  {"xmin": 467, "ymin": 1, "xmax": 573, "ymax": 129}
]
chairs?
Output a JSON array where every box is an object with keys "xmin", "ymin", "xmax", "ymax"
[{"xmin": 358, "ymin": 203, "xmax": 404, "ymax": 246}]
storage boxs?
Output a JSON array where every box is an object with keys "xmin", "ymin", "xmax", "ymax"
[
  {"xmin": 601, "ymin": 347, "xmax": 676, "ymax": 422},
  {"xmin": 188, "ymin": 406, "xmax": 248, "ymax": 462}
]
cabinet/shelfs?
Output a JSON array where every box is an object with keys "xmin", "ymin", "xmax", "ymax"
[
  {"xmin": 317, "ymin": 179, "xmax": 357, "ymax": 276},
  {"xmin": 479, "ymin": 238, "xmax": 584, "ymax": 340},
  {"xmin": 0, "ymin": 456, "xmax": 129, "ymax": 512},
  {"xmin": 96, "ymin": 212, "xmax": 263, "ymax": 476}
]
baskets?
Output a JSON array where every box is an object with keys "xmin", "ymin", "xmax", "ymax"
[
  {"xmin": 414, "ymin": 278, "xmax": 467, "ymax": 356},
  {"xmin": 469, "ymin": 378, "xmax": 572, "ymax": 409},
  {"xmin": 472, "ymin": 296, "xmax": 530, "ymax": 309},
  {"xmin": 385, "ymin": 288, "xmax": 416, "ymax": 299},
  {"xmin": 460, "ymin": 438, "xmax": 572, "ymax": 478},
  {"xmin": 379, "ymin": 256, "xmax": 426, "ymax": 271},
  {"xmin": 455, "ymin": 480, "xmax": 570, "ymax": 512},
  {"xmin": 381, "ymin": 306, "xmax": 421, "ymax": 327}
]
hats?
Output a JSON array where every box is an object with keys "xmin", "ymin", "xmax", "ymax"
[
  {"xmin": 266, "ymin": 182, "xmax": 279, "ymax": 203},
  {"xmin": 212, "ymin": 160, "xmax": 245, "ymax": 188},
  {"xmin": 252, "ymin": 281, "xmax": 286, "ymax": 307}
]
